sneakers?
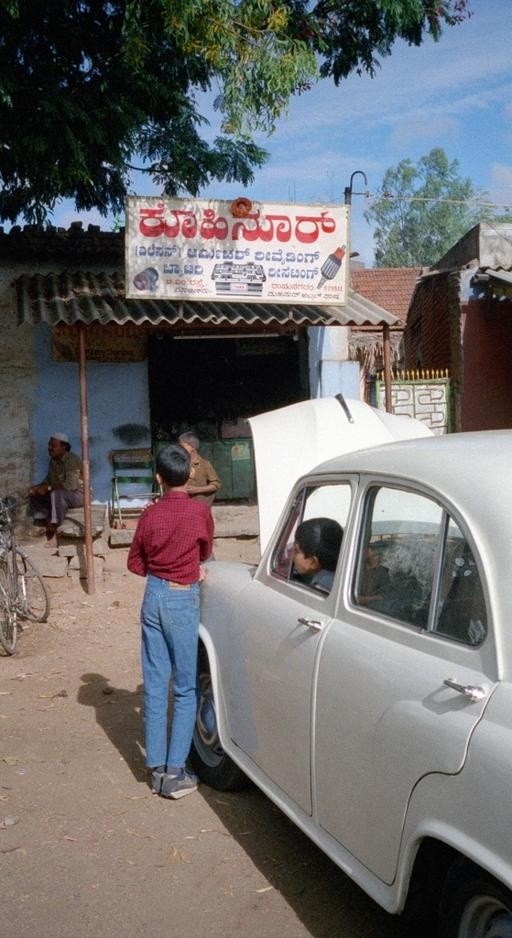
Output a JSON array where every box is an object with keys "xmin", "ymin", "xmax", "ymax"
[
  {"xmin": 151, "ymin": 770, "xmax": 164, "ymax": 793},
  {"xmin": 160, "ymin": 767, "xmax": 199, "ymax": 800}
]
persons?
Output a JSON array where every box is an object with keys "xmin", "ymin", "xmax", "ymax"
[
  {"xmin": 27, "ymin": 432, "xmax": 96, "ymax": 548},
  {"xmin": 289, "ymin": 516, "xmax": 383, "ymax": 602},
  {"xmin": 128, "ymin": 444, "xmax": 216, "ymax": 802},
  {"xmin": 156, "ymin": 431, "xmax": 222, "ymax": 566}
]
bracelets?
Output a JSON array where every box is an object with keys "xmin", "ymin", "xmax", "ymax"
[{"xmin": 47, "ymin": 484, "xmax": 52, "ymax": 490}]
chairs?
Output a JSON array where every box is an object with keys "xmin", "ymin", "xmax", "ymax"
[{"xmin": 107, "ymin": 446, "xmax": 161, "ymax": 531}]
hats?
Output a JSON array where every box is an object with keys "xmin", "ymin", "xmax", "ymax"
[{"xmin": 51, "ymin": 432, "xmax": 72, "ymax": 448}]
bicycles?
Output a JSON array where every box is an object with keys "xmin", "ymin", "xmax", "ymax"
[{"xmin": 0, "ymin": 499, "xmax": 50, "ymax": 655}]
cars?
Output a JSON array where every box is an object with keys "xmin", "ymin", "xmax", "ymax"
[{"xmin": 188, "ymin": 394, "xmax": 512, "ymax": 938}]
